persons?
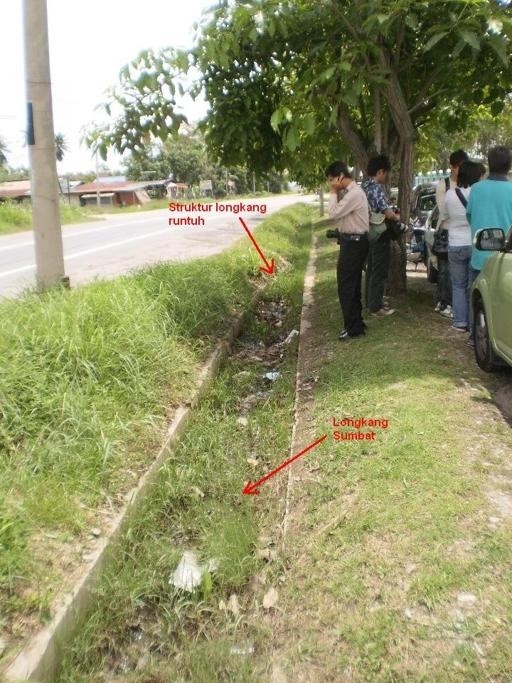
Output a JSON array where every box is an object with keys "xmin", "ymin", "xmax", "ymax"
[
  {"xmin": 463, "ymin": 144, "xmax": 511, "ymax": 347},
  {"xmin": 433, "ymin": 147, "xmax": 468, "ymax": 317},
  {"xmin": 440, "ymin": 161, "xmax": 487, "ymax": 333},
  {"xmin": 324, "ymin": 159, "xmax": 371, "ymax": 340},
  {"xmin": 361, "ymin": 152, "xmax": 409, "ymax": 316}
]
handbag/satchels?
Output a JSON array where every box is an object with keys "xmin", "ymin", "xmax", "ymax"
[
  {"xmin": 367, "ymin": 212, "xmax": 387, "ymax": 244},
  {"xmin": 429, "ymin": 230, "xmax": 448, "ymax": 257}
]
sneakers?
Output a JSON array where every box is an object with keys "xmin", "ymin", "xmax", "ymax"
[
  {"xmin": 371, "ymin": 293, "xmax": 396, "ymax": 317},
  {"xmin": 434, "ymin": 302, "xmax": 468, "ymax": 334},
  {"xmin": 339, "ymin": 325, "xmax": 367, "ymax": 341}
]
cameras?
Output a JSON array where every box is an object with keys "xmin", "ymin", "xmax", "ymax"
[
  {"xmin": 325, "ymin": 227, "xmax": 340, "ymax": 243},
  {"xmin": 391, "ymin": 205, "xmax": 410, "ymax": 233}
]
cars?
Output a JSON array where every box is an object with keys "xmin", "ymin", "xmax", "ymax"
[
  {"xmin": 390, "ymin": 184, "xmax": 437, "ymax": 282},
  {"xmin": 470, "ymin": 223, "xmax": 511, "ymax": 374}
]
